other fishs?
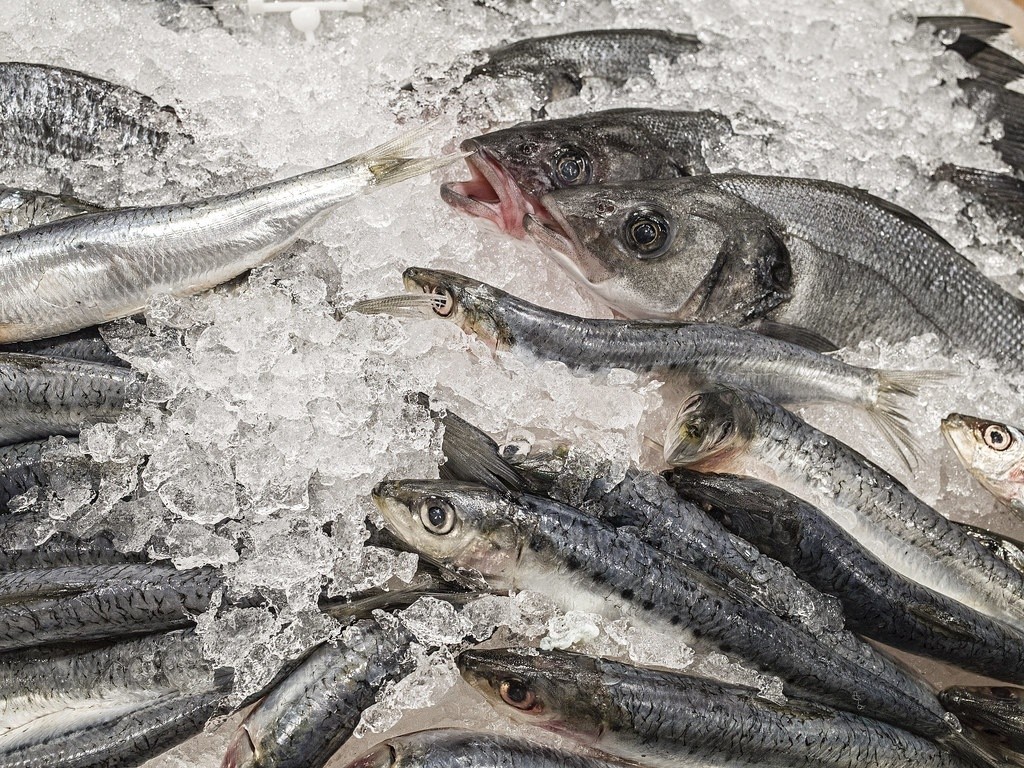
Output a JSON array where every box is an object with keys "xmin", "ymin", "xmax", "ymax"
[{"xmin": 0, "ymin": 0, "xmax": 1022, "ymax": 768}]
